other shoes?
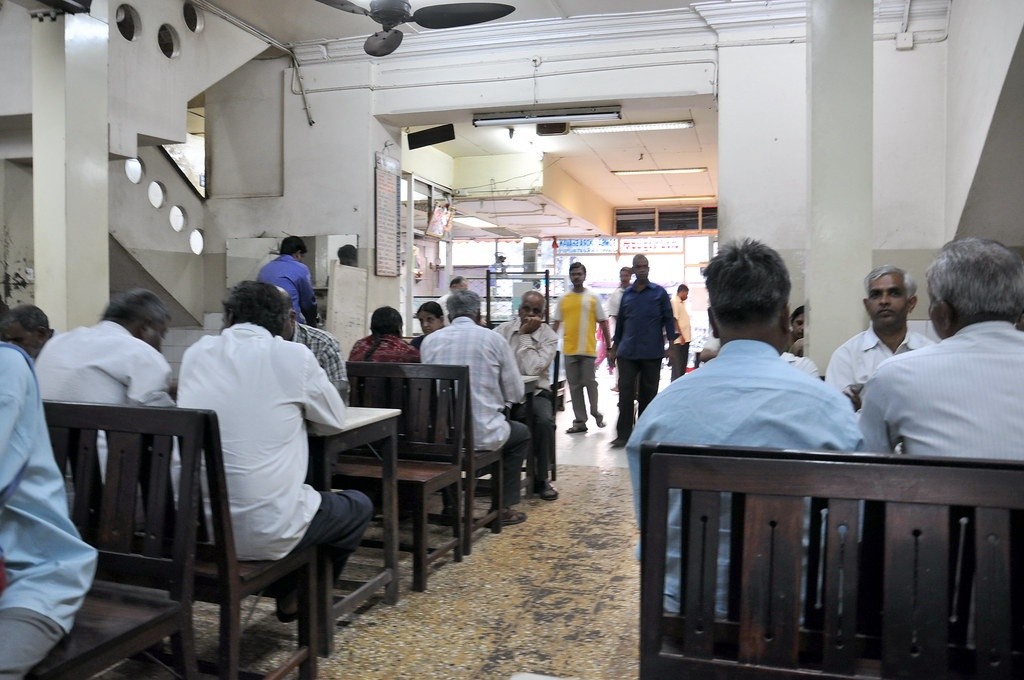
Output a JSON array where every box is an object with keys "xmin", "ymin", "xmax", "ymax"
[
  {"xmin": 611, "ymin": 436, "xmax": 628, "ymax": 446},
  {"xmin": 597, "ymin": 419, "xmax": 606, "ymax": 428},
  {"xmin": 567, "ymin": 425, "xmax": 588, "ymax": 433}
]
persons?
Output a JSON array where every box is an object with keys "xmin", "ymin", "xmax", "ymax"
[
  {"xmin": 33, "ymin": 287, "xmax": 177, "ymax": 486},
  {"xmin": 256, "ymin": 235, "xmax": 319, "ymax": 328},
  {"xmin": 349, "ymin": 306, "xmax": 421, "ymax": 363},
  {"xmin": 410, "ymin": 300, "xmax": 447, "ymax": 353},
  {"xmin": 623, "ymin": 238, "xmax": 865, "ymax": 668},
  {"xmin": 433, "ymin": 277, "xmax": 488, "ymax": 328},
  {"xmin": 551, "ymin": 262, "xmax": 610, "ymax": 434},
  {"xmin": 177, "ymin": 280, "xmax": 373, "ymax": 623},
  {"xmin": 604, "ymin": 266, "xmax": 636, "ymax": 392},
  {"xmin": 338, "ymin": 245, "xmax": 358, "ymax": 267},
  {"xmin": 860, "ymin": 236, "xmax": 1024, "ymax": 457},
  {"xmin": 609, "ymin": 254, "xmax": 682, "ymax": 448},
  {"xmin": 701, "ymin": 336, "xmax": 723, "ymax": 362},
  {"xmin": 823, "ymin": 263, "xmax": 938, "ymax": 423},
  {"xmin": 422, "ymin": 288, "xmax": 527, "ymax": 525},
  {"xmin": 790, "ymin": 302, "xmax": 804, "ymax": 358},
  {"xmin": 1, "ymin": 297, "xmax": 98, "ymax": 680},
  {"xmin": 491, "ymin": 290, "xmax": 561, "ymax": 499},
  {"xmin": 671, "ymin": 284, "xmax": 691, "ymax": 384}
]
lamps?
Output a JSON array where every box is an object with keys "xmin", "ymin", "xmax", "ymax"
[
  {"xmin": 570, "ymin": 119, "xmax": 694, "ymax": 133},
  {"xmin": 638, "ymin": 196, "xmax": 715, "ymax": 202},
  {"xmin": 611, "ymin": 167, "xmax": 707, "ymax": 175},
  {"xmin": 472, "ymin": 105, "xmax": 622, "ymax": 127}
]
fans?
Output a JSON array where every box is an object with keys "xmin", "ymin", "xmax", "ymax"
[{"xmin": 317, "ymin": 0, "xmax": 516, "ymax": 57}]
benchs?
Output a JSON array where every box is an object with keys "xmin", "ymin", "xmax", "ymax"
[
  {"xmin": 0, "ymin": 345, "xmax": 563, "ymax": 680},
  {"xmin": 636, "ymin": 443, "xmax": 1024, "ymax": 680}
]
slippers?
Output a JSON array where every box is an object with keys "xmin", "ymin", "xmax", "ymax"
[{"xmin": 276, "ymin": 594, "xmax": 299, "ymax": 623}]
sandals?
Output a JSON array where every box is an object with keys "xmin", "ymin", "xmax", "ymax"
[
  {"xmin": 484, "ymin": 507, "xmax": 526, "ymax": 525},
  {"xmin": 534, "ymin": 481, "xmax": 557, "ymax": 501}
]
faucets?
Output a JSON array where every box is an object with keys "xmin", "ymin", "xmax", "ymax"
[{"xmin": 316, "ymin": 311, "xmax": 324, "ymax": 327}]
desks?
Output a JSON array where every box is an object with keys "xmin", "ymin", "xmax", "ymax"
[
  {"xmin": 302, "ymin": 407, "xmax": 402, "ymax": 660},
  {"xmin": 520, "ymin": 375, "xmax": 540, "ymax": 395}
]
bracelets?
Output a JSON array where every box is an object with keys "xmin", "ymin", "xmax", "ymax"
[{"xmin": 607, "ymin": 348, "xmax": 612, "ymax": 351}]
